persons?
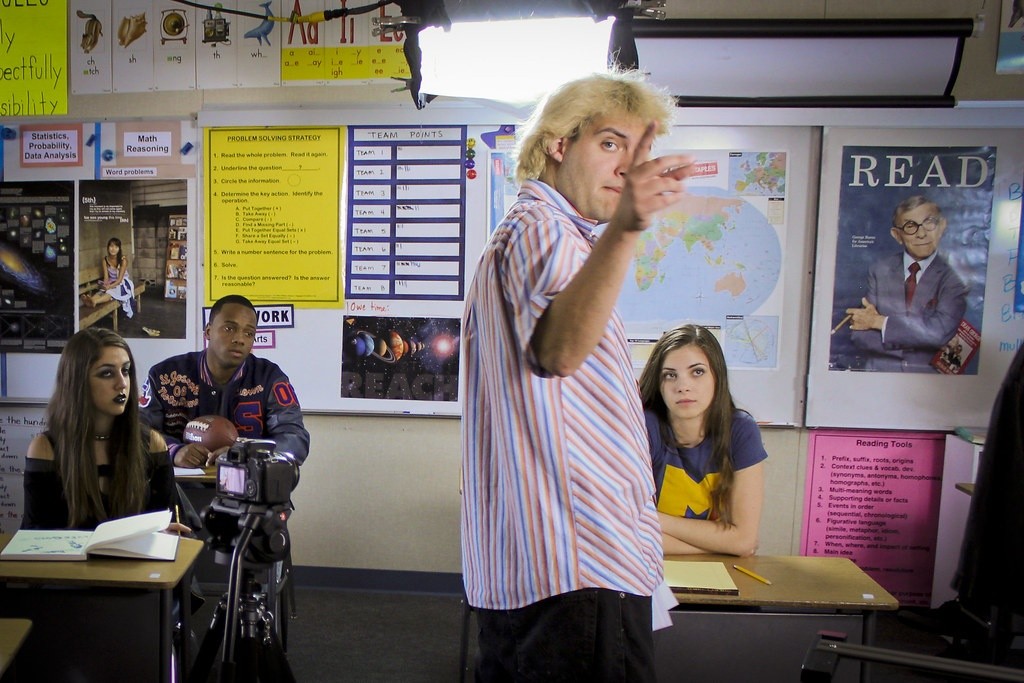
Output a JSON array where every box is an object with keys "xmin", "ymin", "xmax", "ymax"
[
  {"xmin": 844, "ymin": 195, "xmax": 973, "ymax": 376},
  {"xmin": 453, "ymin": 74, "xmax": 696, "ymax": 683},
  {"xmin": 22, "ymin": 326, "xmax": 194, "ymax": 593},
  {"xmin": 138, "ymin": 295, "xmax": 311, "ymax": 515},
  {"xmin": 79, "ymin": 238, "xmax": 138, "ymax": 319},
  {"xmin": 636, "ymin": 322, "xmax": 768, "ymax": 559}
]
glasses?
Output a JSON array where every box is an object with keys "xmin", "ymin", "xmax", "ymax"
[{"xmin": 895, "ymin": 217, "xmax": 940, "ymax": 235}]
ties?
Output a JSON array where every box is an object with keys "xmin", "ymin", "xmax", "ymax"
[{"xmin": 904, "ymin": 262, "xmax": 920, "ymax": 317}]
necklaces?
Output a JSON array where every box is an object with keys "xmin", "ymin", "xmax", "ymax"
[{"xmin": 89, "ymin": 432, "xmax": 113, "ymax": 441}]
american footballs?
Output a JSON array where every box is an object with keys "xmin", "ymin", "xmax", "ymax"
[{"xmin": 183, "ymin": 416, "xmax": 240, "ymax": 451}]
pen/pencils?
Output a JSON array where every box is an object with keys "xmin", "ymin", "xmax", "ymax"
[
  {"xmin": 733, "ymin": 564, "xmax": 773, "ymax": 587},
  {"xmin": 830, "ymin": 310, "xmax": 852, "ymax": 336},
  {"xmin": 175, "ymin": 504, "xmax": 182, "ymax": 537}
]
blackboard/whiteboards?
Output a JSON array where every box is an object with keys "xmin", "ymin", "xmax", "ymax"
[{"xmin": 0, "ymin": 407, "xmax": 51, "ymax": 534}]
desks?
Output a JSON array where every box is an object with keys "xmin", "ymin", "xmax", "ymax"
[
  {"xmin": 173, "ymin": 463, "xmax": 283, "ymax": 655},
  {"xmin": 0, "ymin": 532, "xmax": 207, "ymax": 683},
  {"xmin": 649, "ymin": 555, "xmax": 899, "ymax": 682}
]
